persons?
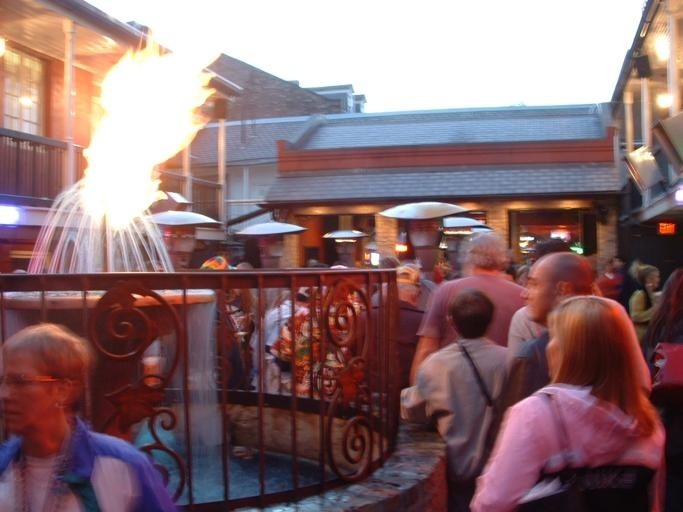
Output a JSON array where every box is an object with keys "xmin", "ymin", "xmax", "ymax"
[
  {"xmin": 595, "ymin": 255, "xmax": 683, "ymax": 511},
  {"xmin": 472, "ymin": 253, "xmax": 594, "ymax": 472},
  {"xmin": 0, "ymin": 322, "xmax": 177, "ymax": 510},
  {"xmin": 469, "ymin": 295, "xmax": 665, "ymax": 512},
  {"xmin": 199, "ymin": 256, "xmax": 359, "ymax": 401},
  {"xmin": 371, "ymin": 228, "xmax": 573, "ymax": 387},
  {"xmin": 398, "ymin": 288, "xmax": 511, "ymax": 511}
]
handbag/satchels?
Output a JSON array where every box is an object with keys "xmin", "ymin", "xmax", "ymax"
[{"xmin": 507, "ymin": 391, "xmax": 659, "ymax": 512}]
